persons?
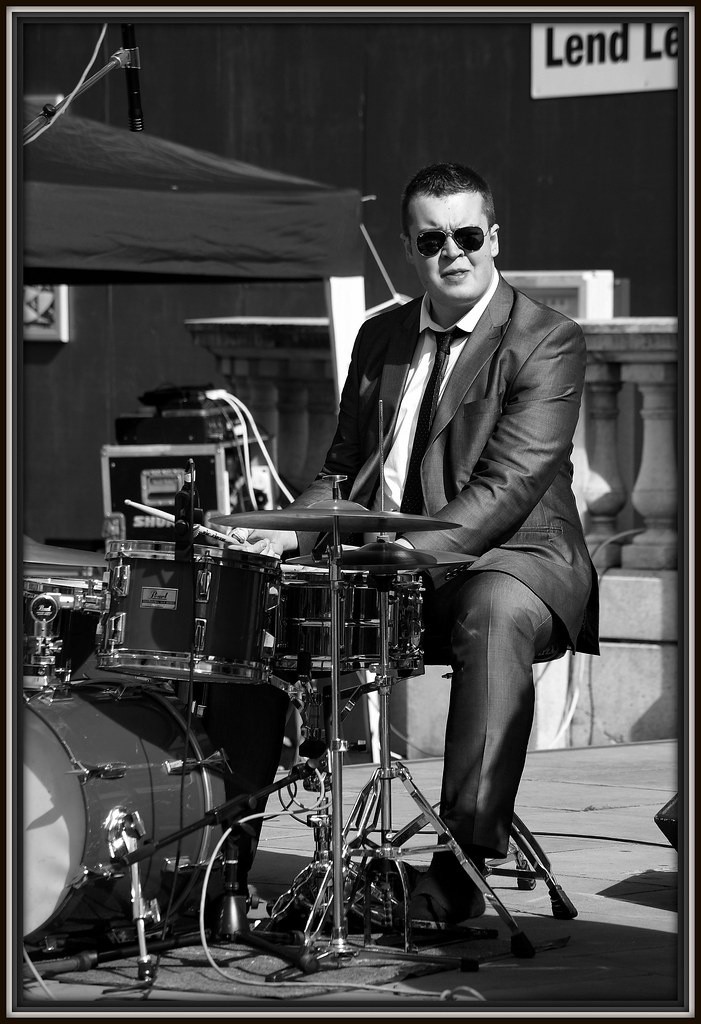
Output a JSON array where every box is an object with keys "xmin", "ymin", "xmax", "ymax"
[{"xmin": 207, "ymin": 163, "xmax": 603, "ymax": 926}]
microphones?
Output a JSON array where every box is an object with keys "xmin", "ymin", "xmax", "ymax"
[
  {"xmin": 122, "ymin": 23, "xmax": 145, "ymax": 131},
  {"xmin": 183, "ymin": 458, "xmax": 204, "ymax": 524}
]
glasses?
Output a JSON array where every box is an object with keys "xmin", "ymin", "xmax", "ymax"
[{"xmin": 415, "ymin": 225, "xmax": 485, "ymax": 259}]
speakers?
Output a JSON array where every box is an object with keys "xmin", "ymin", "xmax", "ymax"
[{"xmin": 654, "ymin": 793, "xmax": 679, "ymax": 853}]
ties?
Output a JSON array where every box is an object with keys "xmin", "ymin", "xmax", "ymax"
[{"xmin": 395, "ymin": 326, "xmax": 471, "ymax": 540}]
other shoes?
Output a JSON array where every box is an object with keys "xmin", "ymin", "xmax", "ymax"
[{"xmin": 395, "ymin": 884, "xmax": 485, "ymax": 922}]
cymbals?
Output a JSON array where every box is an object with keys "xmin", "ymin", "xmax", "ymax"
[
  {"xmin": 207, "ymin": 499, "xmax": 479, "ymax": 566},
  {"xmin": 23, "ymin": 534, "xmax": 113, "ymax": 567}
]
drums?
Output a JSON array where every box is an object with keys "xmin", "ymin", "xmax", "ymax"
[
  {"xmin": 95, "ymin": 539, "xmax": 281, "ymax": 685},
  {"xmin": 17, "ymin": 574, "xmax": 148, "ymax": 690},
  {"xmin": 275, "ymin": 564, "xmax": 424, "ymax": 672},
  {"xmin": 18, "ymin": 678, "xmax": 212, "ymax": 950}
]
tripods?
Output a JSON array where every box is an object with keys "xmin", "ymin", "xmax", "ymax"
[{"xmin": 71, "ymin": 518, "xmax": 535, "ymax": 983}]
